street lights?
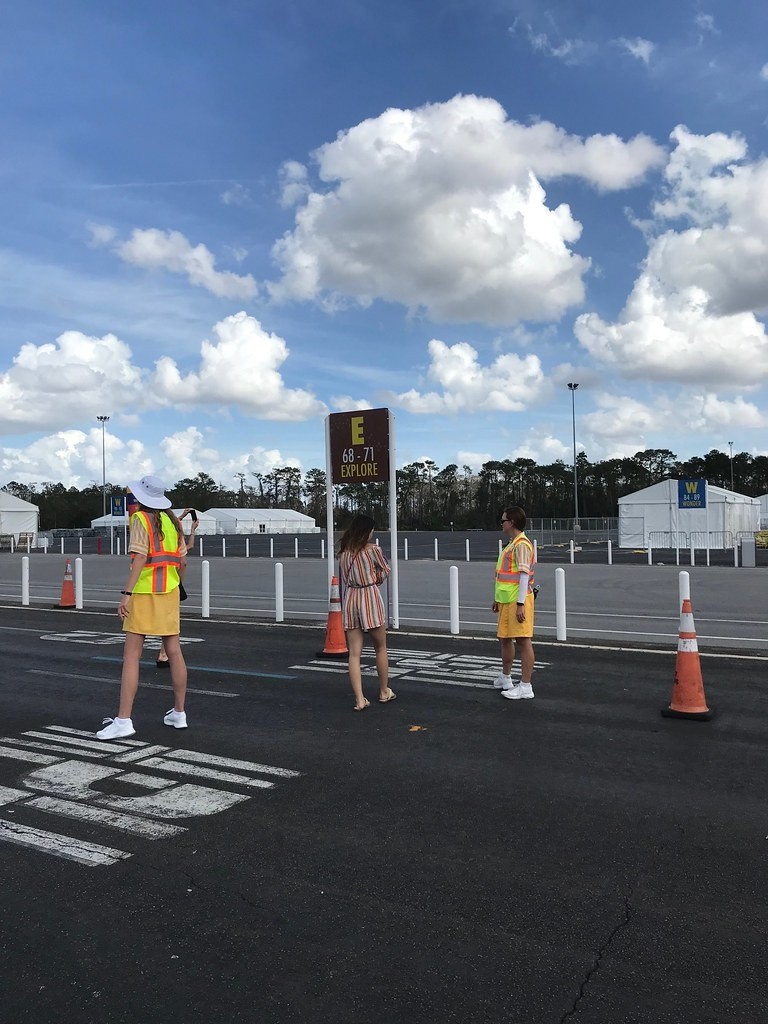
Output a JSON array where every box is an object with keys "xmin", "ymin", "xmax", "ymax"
[
  {"xmin": 728, "ymin": 441, "xmax": 734, "ymax": 492},
  {"xmin": 96, "ymin": 415, "xmax": 111, "ymax": 515},
  {"xmin": 566, "ymin": 383, "xmax": 581, "ymax": 532}
]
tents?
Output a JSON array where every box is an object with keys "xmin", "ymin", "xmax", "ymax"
[
  {"xmin": 91, "ymin": 508, "xmax": 216, "ymax": 535},
  {"xmin": 0, "ymin": 490, "xmax": 39, "ymax": 535},
  {"xmin": 618, "ymin": 479, "xmax": 762, "ymax": 548},
  {"xmin": 203, "ymin": 508, "xmax": 315, "ymax": 534}
]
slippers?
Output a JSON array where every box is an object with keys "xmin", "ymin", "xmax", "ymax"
[
  {"xmin": 353, "ymin": 697, "xmax": 372, "ymax": 710},
  {"xmin": 379, "ymin": 687, "xmax": 397, "ymax": 702}
]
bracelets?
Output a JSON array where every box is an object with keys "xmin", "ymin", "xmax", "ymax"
[
  {"xmin": 121, "ymin": 591, "xmax": 132, "ymax": 596},
  {"xmin": 517, "ymin": 602, "xmax": 524, "ymax": 606}
]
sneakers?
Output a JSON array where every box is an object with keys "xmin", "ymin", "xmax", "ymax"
[
  {"xmin": 493, "ymin": 671, "xmax": 513, "ymax": 689},
  {"xmin": 164, "ymin": 708, "xmax": 188, "ymax": 728},
  {"xmin": 96, "ymin": 716, "xmax": 136, "ymax": 740},
  {"xmin": 501, "ymin": 680, "xmax": 535, "ymax": 700}
]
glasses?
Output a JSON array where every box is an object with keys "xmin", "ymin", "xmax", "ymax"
[{"xmin": 501, "ymin": 519, "xmax": 512, "ymax": 524}]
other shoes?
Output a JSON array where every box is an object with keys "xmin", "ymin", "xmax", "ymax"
[{"xmin": 156, "ymin": 658, "xmax": 171, "ymax": 667}]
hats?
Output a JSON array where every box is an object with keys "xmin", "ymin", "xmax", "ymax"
[{"xmin": 128, "ymin": 476, "xmax": 172, "ymax": 509}]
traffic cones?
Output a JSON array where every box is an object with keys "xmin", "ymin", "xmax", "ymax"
[
  {"xmin": 52, "ymin": 559, "xmax": 75, "ymax": 609},
  {"xmin": 316, "ymin": 576, "xmax": 350, "ymax": 660},
  {"xmin": 660, "ymin": 598, "xmax": 718, "ymax": 721}
]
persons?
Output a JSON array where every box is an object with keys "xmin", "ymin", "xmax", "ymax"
[
  {"xmin": 156, "ymin": 509, "xmax": 200, "ymax": 668},
  {"xmin": 492, "ymin": 507, "xmax": 535, "ymax": 699},
  {"xmin": 335, "ymin": 514, "xmax": 397, "ymax": 711},
  {"xmin": 96, "ymin": 476, "xmax": 188, "ymax": 739}
]
